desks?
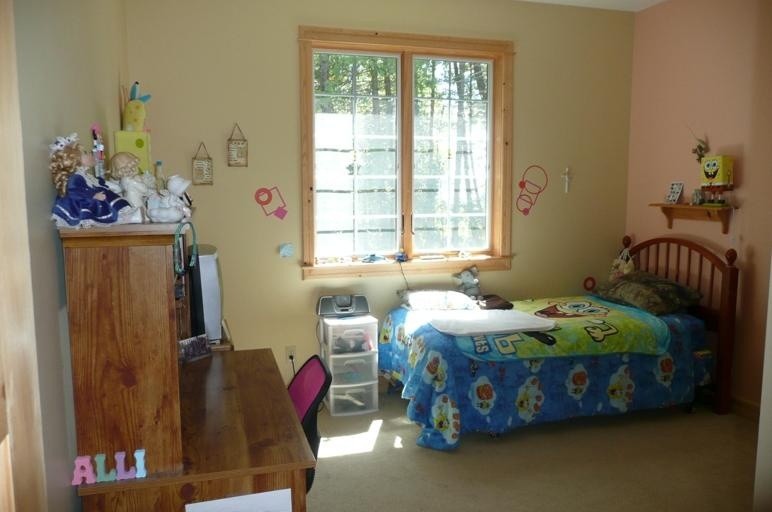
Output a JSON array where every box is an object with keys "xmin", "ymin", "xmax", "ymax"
[{"xmin": 71, "ymin": 348, "xmax": 315, "ymax": 512}]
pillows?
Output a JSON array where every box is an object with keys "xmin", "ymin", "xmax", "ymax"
[{"xmin": 592, "ymin": 268, "xmax": 705, "ymax": 315}]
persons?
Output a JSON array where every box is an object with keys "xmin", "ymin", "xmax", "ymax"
[{"xmin": 47, "ymin": 123, "xmax": 193, "ymax": 231}]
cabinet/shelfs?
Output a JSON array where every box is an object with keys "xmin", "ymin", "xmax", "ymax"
[{"xmin": 58, "ymin": 216, "xmax": 188, "ymax": 482}]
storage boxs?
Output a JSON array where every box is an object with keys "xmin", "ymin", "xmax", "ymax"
[{"xmin": 319, "ymin": 313, "xmax": 379, "ymax": 417}]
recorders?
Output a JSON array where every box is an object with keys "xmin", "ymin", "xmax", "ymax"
[{"xmin": 315, "ymin": 294, "xmax": 370, "ymax": 316}]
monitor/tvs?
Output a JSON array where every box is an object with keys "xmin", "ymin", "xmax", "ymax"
[{"xmin": 189, "ymin": 244, "xmax": 225, "ymax": 340}]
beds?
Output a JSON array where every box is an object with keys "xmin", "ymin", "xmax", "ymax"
[{"xmin": 378, "ymin": 235, "xmax": 737, "ymax": 437}]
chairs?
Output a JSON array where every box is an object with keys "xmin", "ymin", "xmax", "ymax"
[{"xmin": 287, "ymin": 356, "xmax": 331, "ymax": 492}]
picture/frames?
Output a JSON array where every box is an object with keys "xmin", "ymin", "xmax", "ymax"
[{"xmin": 667, "ymin": 182, "xmax": 683, "ymax": 203}]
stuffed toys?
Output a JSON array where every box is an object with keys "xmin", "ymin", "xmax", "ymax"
[
  {"xmin": 123, "ymin": 79, "xmax": 152, "ymax": 131},
  {"xmin": 697, "ymin": 154, "xmax": 734, "ymax": 207},
  {"xmin": 452, "ymin": 266, "xmax": 484, "ymax": 301}
]
